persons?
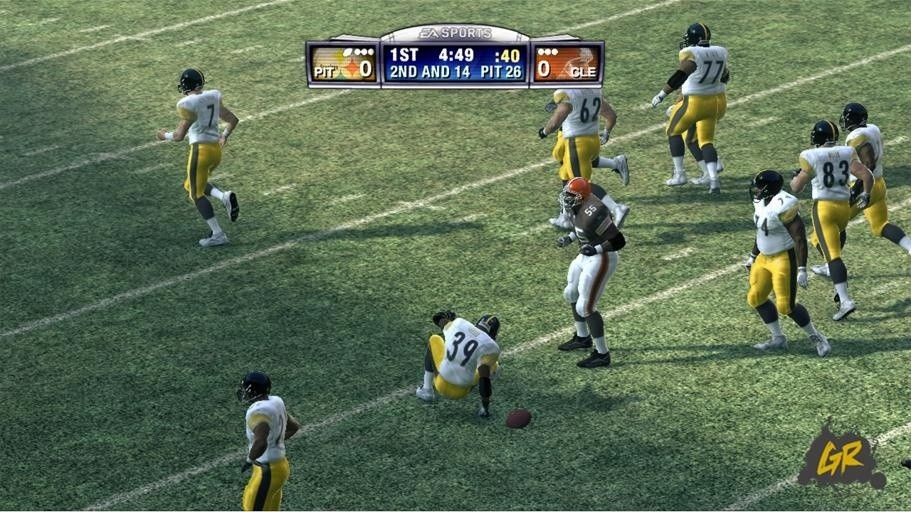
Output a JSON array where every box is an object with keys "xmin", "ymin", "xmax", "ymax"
[
  {"xmin": 666, "ymin": 41, "xmax": 727, "ymax": 186},
  {"xmin": 651, "ymin": 22, "xmax": 730, "ymax": 194},
  {"xmin": 556, "ymin": 177, "xmax": 627, "ymax": 369},
  {"xmin": 545, "ymin": 99, "xmax": 629, "ymax": 185},
  {"xmin": 416, "ymin": 308, "xmax": 501, "ymax": 418},
  {"xmin": 236, "ymin": 369, "xmax": 301, "ymax": 511},
  {"xmin": 539, "ymin": 89, "xmax": 630, "ymax": 230},
  {"xmin": 158, "ymin": 67, "xmax": 240, "ymax": 247},
  {"xmin": 790, "ymin": 120, "xmax": 875, "ymax": 321},
  {"xmin": 809, "ymin": 102, "xmax": 911, "ymax": 278},
  {"xmin": 747, "ymin": 170, "xmax": 831, "ymax": 358}
]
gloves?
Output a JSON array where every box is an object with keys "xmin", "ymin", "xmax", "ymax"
[
  {"xmin": 446, "ymin": 310, "xmax": 456, "ymax": 319},
  {"xmin": 555, "ymin": 234, "xmax": 596, "ymax": 257},
  {"xmin": 480, "ymin": 408, "xmax": 489, "ymax": 416},
  {"xmin": 241, "ymin": 463, "xmax": 252, "ymax": 472}
]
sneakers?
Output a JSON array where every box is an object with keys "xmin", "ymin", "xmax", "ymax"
[
  {"xmin": 199, "ymin": 233, "xmax": 228, "ymax": 246},
  {"xmin": 613, "ymin": 204, "xmax": 630, "ymax": 228},
  {"xmin": 416, "ymin": 384, "xmax": 435, "ymax": 402},
  {"xmin": 550, "ymin": 217, "xmax": 574, "ymax": 231},
  {"xmin": 666, "ymin": 163, "xmax": 723, "ymax": 194},
  {"xmin": 614, "ymin": 155, "xmax": 629, "ymax": 186},
  {"xmin": 577, "ymin": 350, "xmax": 611, "ymax": 368},
  {"xmin": 221, "ymin": 191, "xmax": 241, "ymax": 223},
  {"xmin": 756, "ymin": 263, "xmax": 857, "ymax": 354},
  {"xmin": 558, "ymin": 331, "xmax": 593, "ymax": 351}
]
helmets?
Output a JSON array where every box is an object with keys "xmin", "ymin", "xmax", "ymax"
[
  {"xmin": 840, "ymin": 102, "xmax": 868, "ymax": 133},
  {"xmin": 236, "ymin": 370, "xmax": 271, "ymax": 407},
  {"xmin": 177, "ymin": 68, "xmax": 206, "ymax": 96},
  {"xmin": 475, "ymin": 315, "xmax": 499, "ymax": 339},
  {"xmin": 811, "ymin": 120, "xmax": 839, "ymax": 148},
  {"xmin": 680, "ymin": 23, "xmax": 711, "ymax": 49}
]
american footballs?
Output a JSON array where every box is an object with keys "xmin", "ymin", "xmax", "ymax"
[{"xmin": 506, "ymin": 409, "xmax": 533, "ymax": 428}]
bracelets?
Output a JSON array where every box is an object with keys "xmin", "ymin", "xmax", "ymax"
[
  {"xmin": 165, "ymin": 132, "xmax": 174, "ymax": 141},
  {"xmin": 223, "ymin": 129, "xmax": 231, "ymax": 138}
]
